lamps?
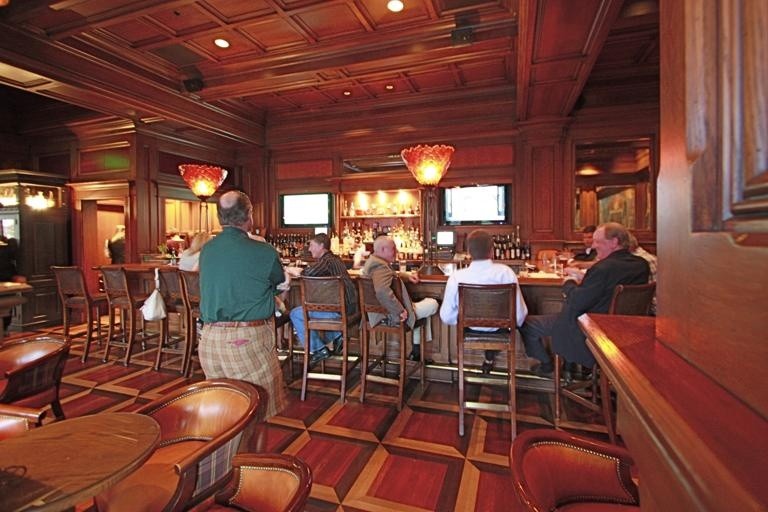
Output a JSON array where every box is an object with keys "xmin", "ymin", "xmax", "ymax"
[
  {"xmin": 401, "ymin": 144, "xmax": 456, "ymax": 276},
  {"xmin": 179, "ymin": 161, "xmax": 228, "ymax": 234}
]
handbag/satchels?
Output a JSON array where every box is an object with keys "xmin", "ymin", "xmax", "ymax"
[{"xmin": 137, "ymin": 267, "xmax": 167, "ymax": 322}]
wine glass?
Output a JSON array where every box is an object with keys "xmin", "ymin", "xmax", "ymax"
[{"xmin": 282, "ymin": 258, "xmax": 290, "ymax": 268}]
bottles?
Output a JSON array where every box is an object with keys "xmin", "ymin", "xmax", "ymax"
[
  {"xmin": 491, "ymin": 224, "xmax": 531, "ymax": 260},
  {"xmin": 342, "ymin": 199, "xmax": 420, "ymax": 217},
  {"xmin": 170, "ymin": 247, "xmax": 177, "ymax": 266},
  {"xmin": 330, "ymin": 230, "xmax": 340, "ymax": 252},
  {"xmin": 265, "ymin": 231, "xmax": 318, "ymax": 256},
  {"xmin": 341, "ymin": 220, "xmax": 424, "ymax": 260}
]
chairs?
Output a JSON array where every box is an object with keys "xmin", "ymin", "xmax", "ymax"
[
  {"xmin": 458, "ymin": 283, "xmax": 516, "ymax": 442},
  {"xmin": 208, "ymin": 453, "xmax": 313, "ymax": 512},
  {"xmin": 0, "ymin": 406, "xmax": 48, "ymax": 441},
  {"xmin": 355, "ymin": 277, "xmax": 426, "ymax": 411},
  {"xmin": 49, "ymin": 264, "xmax": 108, "ymax": 363},
  {"xmin": 153, "ymin": 267, "xmax": 188, "ymax": 375},
  {"xmin": 510, "ymin": 428, "xmax": 639, "ymax": 511},
  {"xmin": 552, "ymin": 280, "xmax": 655, "ymax": 445},
  {"xmin": 90, "ymin": 264, "xmax": 151, "ymax": 366},
  {"xmin": 180, "ymin": 270, "xmax": 205, "ymax": 379},
  {"xmin": 299, "ymin": 276, "xmax": 361, "ymax": 403},
  {"xmin": 95, "ymin": 377, "xmax": 260, "ymax": 512},
  {"xmin": 1, "ymin": 330, "xmax": 71, "ymax": 420}
]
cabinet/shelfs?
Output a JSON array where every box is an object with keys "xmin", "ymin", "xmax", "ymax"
[{"xmin": 338, "ymin": 187, "xmax": 424, "ymax": 251}]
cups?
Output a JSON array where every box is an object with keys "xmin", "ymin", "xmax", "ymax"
[
  {"xmin": 545, "ymin": 253, "xmax": 567, "ymax": 278},
  {"xmin": 399, "ymin": 259, "xmax": 407, "ymax": 272},
  {"xmin": 296, "ymin": 260, "xmax": 302, "ymax": 268},
  {"xmin": 411, "ymin": 266, "xmax": 418, "ymax": 275},
  {"xmin": 518, "ymin": 266, "xmax": 529, "ymax": 281}
]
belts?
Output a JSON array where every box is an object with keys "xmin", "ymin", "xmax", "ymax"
[{"xmin": 201, "ymin": 320, "xmax": 271, "ymax": 329}]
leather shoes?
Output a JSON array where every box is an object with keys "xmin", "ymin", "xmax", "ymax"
[
  {"xmin": 560, "ymin": 364, "xmax": 576, "ymax": 386},
  {"xmin": 535, "ymin": 363, "xmax": 554, "ymax": 378},
  {"xmin": 334, "ymin": 335, "xmax": 349, "ymax": 355},
  {"xmin": 408, "ymin": 352, "xmax": 433, "ymax": 365},
  {"xmin": 482, "ymin": 362, "xmax": 494, "ymax": 374},
  {"xmin": 309, "ymin": 347, "xmax": 331, "ymax": 366}
]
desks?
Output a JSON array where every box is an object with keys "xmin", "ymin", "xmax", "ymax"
[{"xmin": 1, "ymin": 411, "xmax": 161, "ymax": 510}]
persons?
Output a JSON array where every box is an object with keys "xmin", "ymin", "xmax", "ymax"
[
  {"xmin": 107, "ymin": 225, "xmax": 125, "ymax": 265},
  {"xmin": 246, "ymin": 215, "xmax": 286, "ymax": 352},
  {"xmin": 560, "ymin": 225, "xmax": 599, "ymax": 269},
  {"xmin": 289, "ymin": 233, "xmax": 359, "ymax": 370},
  {"xmin": 178, "ymin": 232, "xmax": 213, "ymax": 273},
  {"xmin": 519, "ymin": 222, "xmax": 650, "ymax": 382},
  {"xmin": 364, "ymin": 236, "xmax": 440, "ymax": 366},
  {"xmin": 625, "ymin": 230, "xmax": 657, "ymax": 318},
  {"xmin": 198, "ymin": 190, "xmax": 289, "ymax": 454},
  {"xmin": 438, "ymin": 229, "xmax": 528, "ymax": 374}
]
